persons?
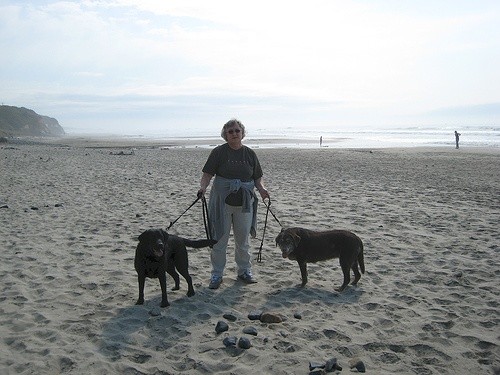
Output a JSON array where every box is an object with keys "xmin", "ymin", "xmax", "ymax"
[
  {"xmin": 320, "ymin": 136, "xmax": 323, "ymax": 146},
  {"xmin": 455, "ymin": 131, "xmax": 461, "ymax": 149},
  {"xmin": 196, "ymin": 120, "xmax": 271, "ymax": 288}
]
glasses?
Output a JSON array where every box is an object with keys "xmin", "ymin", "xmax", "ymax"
[{"xmin": 228, "ymin": 129, "xmax": 241, "ymax": 134}]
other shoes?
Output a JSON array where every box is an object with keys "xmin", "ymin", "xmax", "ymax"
[
  {"xmin": 208, "ymin": 276, "xmax": 223, "ymax": 288},
  {"xmin": 237, "ymin": 272, "xmax": 257, "ymax": 283}
]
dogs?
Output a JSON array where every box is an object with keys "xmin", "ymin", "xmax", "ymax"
[
  {"xmin": 134, "ymin": 227, "xmax": 218, "ymax": 308},
  {"xmin": 275, "ymin": 227, "xmax": 365, "ymax": 292}
]
